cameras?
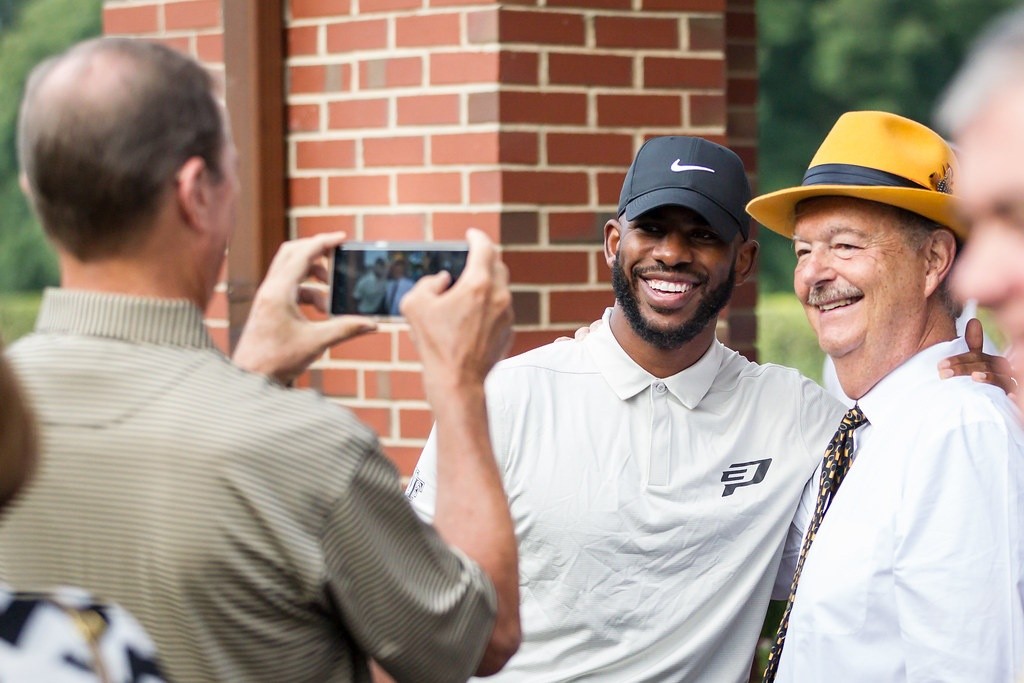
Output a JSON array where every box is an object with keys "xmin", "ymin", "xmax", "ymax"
[{"xmin": 323, "ymin": 240, "xmax": 473, "ymax": 333}]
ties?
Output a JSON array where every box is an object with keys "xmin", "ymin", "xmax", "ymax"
[
  {"xmin": 389, "ymin": 281, "xmax": 400, "ymax": 311},
  {"xmin": 763, "ymin": 406, "xmax": 869, "ymax": 681}
]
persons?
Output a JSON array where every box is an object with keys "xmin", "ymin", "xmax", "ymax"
[
  {"xmin": 751, "ymin": 6, "xmax": 1022, "ymax": 683},
  {"xmin": 0, "ymin": 346, "xmax": 169, "ymax": 681},
  {"xmin": 0, "ymin": 34, "xmax": 521, "ymax": 681},
  {"xmin": 366, "ymin": 136, "xmax": 1018, "ymax": 683},
  {"xmin": 348, "ymin": 256, "xmax": 457, "ymax": 320}
]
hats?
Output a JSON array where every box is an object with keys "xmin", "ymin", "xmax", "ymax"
[
  {"xmin": 745, "ymin": 110, "xmax": 976, "ymax": 243},
  {"xmin": 617, "ymin": 136, "xmax": 752, "ymax": 243}
]
